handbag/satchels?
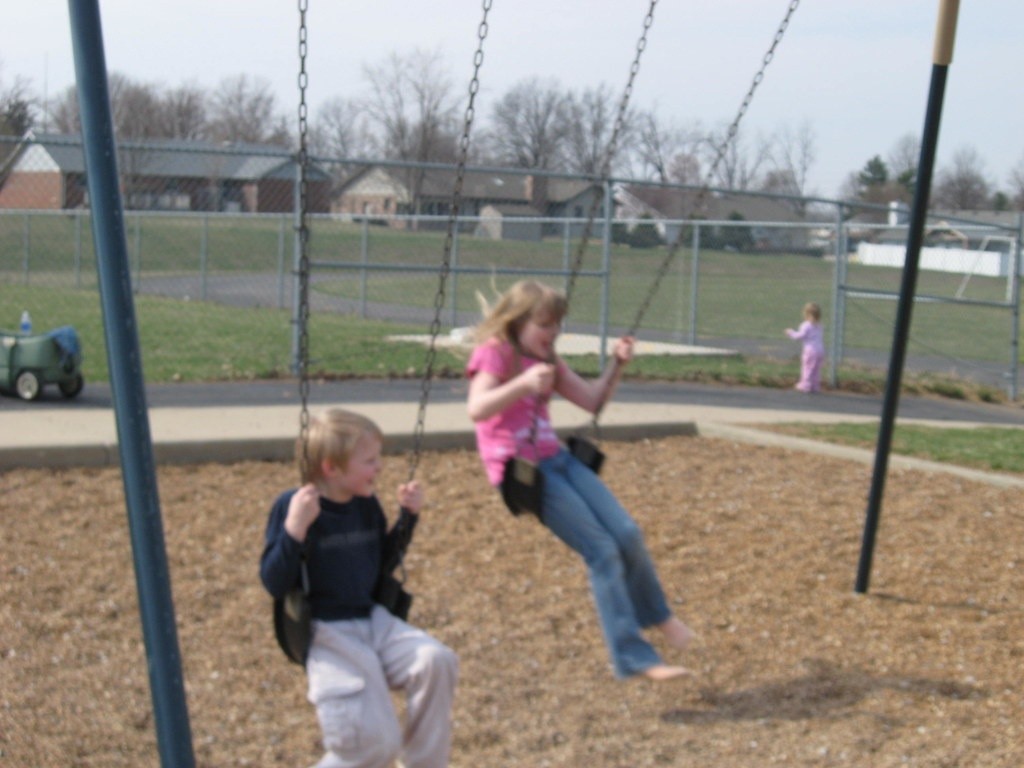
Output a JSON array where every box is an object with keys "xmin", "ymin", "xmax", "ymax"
[{"xmin": 47, "ymin": 325, "xmax": 81, "ymax": 367}]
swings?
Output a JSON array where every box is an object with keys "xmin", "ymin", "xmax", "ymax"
[
  {"xmin": 501, "ymin": 2, "xmax": 800, "ymax": 522},
  {"xmin": 274, "ymin": 0, "xmax": 495, "ymax": 666}
]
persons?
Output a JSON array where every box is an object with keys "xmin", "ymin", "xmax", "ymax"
[
  {"xmin": 785, "ymin": 303, "xmax": 824, "ymax": 393},
  {"xmin": 467, "ymin": 282, "xmax": 696, "ymax": 680},
  {"xmin": 260, "ymin": 409, "xmax": 457, "ymax": 768}
]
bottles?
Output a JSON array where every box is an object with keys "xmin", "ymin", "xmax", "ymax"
[{"xmin": 18, "ymin": 310, "xmax": 33, "ymax": 338}]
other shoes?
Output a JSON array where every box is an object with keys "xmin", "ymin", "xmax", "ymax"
[
  {"xmin": 813, "ymin": 386, "xmax": 820, "ymax": 392},
  {"xmin": 795, "ymin": 383, "xmax": 811, "ymax": 392}
]
